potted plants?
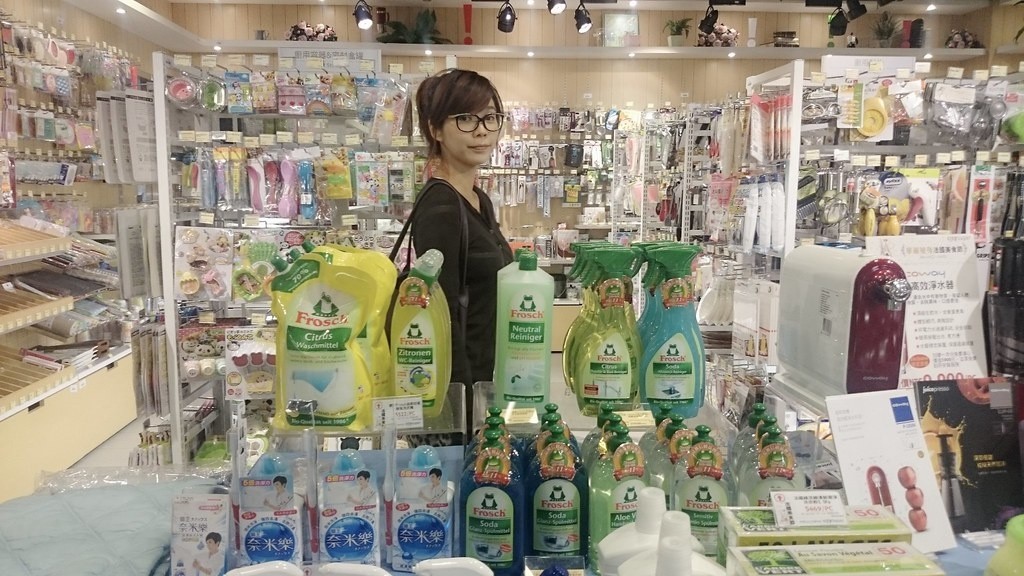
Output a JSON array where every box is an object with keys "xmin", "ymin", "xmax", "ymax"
[
  {"xmin": 662, "ymin": 18, "xmax": 693, "ymax": 47},
  {"xmin": 869, "ymin": 16, "xmax": 902, "ymax": 48}
]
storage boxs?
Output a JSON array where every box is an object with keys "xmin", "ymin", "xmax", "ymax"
[
  {"xmin": 716, "ymin": 505, "xmax": 913, "ymax": 567},
  {"xmin": 725, "ymin": 541, "xmax": 944, "ymax": 576}
]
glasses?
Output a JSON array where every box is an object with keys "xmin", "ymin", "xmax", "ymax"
[{"xmin": 445, "ymin": 110, "xmax": 505, "ymax": 132}]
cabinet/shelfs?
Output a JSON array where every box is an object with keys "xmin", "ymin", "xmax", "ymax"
[{"xmin": 0, "ymin": 215, "xmax": 137, "ymax": 500}]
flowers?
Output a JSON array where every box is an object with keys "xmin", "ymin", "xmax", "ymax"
[
  {"xmin": 284, "ymin": 21, "xmax": 337, "ymax": 41},
  {"xmin": 698, "ymin": 25, "xmax": 741, "ymax": 47},
  {"xmin": 945, "ymin": 29, "xmax": 974, "ymax": 48}
]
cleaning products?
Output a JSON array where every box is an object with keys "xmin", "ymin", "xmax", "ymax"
[
  {"xmin": 169, "ymin": 399, "xmax": 797, "ymax": 576},
  {"xmin": 270, "ymin": 240, "xmax": 705, "ymax": 433}
]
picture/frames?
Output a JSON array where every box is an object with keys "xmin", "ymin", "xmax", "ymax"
[{"xmin": 601, "ymin": 10, "xmax": 641, "ymax": 48}]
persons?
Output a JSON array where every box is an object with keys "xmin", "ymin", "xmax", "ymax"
[
  {"xmin": 347, "ymin": 470, "xmax": 376, "ymax": 506},
  {"xmin": 192, "ymin": 532, "xmax": 224, "ymax": 576},
  {"xmin": 418, "ymin": 468, "xmax": 447, "ymax": 504},
  {"xmin": 264, "ymin": 476, "xmax": 294, "ymax": 511},
  {"xmin": 411, "ymin": 68, "xmax": 514, "ymax": 445}
]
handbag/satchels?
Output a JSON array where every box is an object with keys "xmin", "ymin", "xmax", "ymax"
[{"xmin": 385, "ymin": 179, "xmax": 468, "ymax": 350}]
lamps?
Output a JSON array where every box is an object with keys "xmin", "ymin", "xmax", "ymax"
[
  {"xmin": 574, "ymin": 0, "xmax": 593, "ymax": 33},
  {"xmin": 698, "ymin": 0, "xmax": 719, "ymax": 35},
  {"xmin": 497, "ymin": 0, "xmax": 518, "ymax": 33},
  {"xmin": 548, "ymin": 0, "xmax": 567, "ymax": 15},
  {"xmin": 352, "ymin": 0, "xmax": 373, "ymax": 30},
  {"xmin": 828, "ymin": 7, "xmax": 850, "ymax": 36}
]
